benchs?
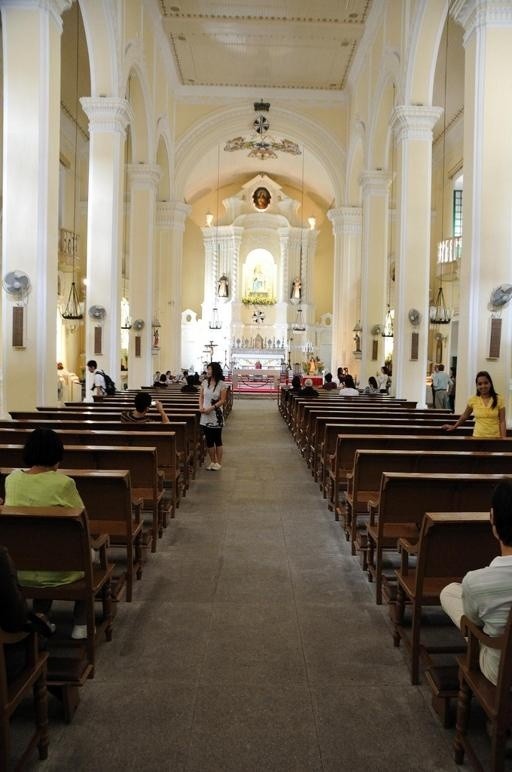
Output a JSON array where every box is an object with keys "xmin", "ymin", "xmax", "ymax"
[
  {"xmin": 279, "ymin": 385, "xmax": 512, "ymax": 772},
  {"xmin": 0, "ymin": 379, "xmax": 233, "ymax": 772}
]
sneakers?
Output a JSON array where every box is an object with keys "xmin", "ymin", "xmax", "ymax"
[
  {"xmin": 72, "ymin": 624, "xmax": 96, "ymax": 639},
  {"xmin": 207, "ymin": 463, "xmax": 213, "ymax": 470},
  {"xmin": 212, "ymin": 463, "xmax": 222, "ymax": 470}
]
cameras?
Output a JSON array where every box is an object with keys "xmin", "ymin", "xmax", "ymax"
[
  {"xmin": 150, "ymin": 400, "xmax": 157, "ymax": 406},
  {"xmin": 344, "ymin": 368, "xmax": 346, "ymax": 370}
]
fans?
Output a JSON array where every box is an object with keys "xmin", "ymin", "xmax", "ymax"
[
  {"xmin": 2, "ymin": 270, "xmax": 31, "ymax": 307},
  {"xmin": 489, "ymin": 282, "xmax": 512, "ymax": 318},
  {"xmin": 408, "ymin": 308, "xmax": 422, "ymax": 332},
  {"xmin": 89, "ymin": 304, "xmax": 106, "ymax": 327},
  {"xmin": 133, "ymin": 320, "xmax": 144, "ymax": 336},
  {"xmin": 370, "ymin": 324, "xmax": 381, "ymax": 340}
]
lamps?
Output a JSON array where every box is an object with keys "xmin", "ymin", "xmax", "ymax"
[
  {"xmin": 61, "ymin": 3, "xmax": 84, "ymax": 327},
  {"xmin": 427, "ymin": 3, "xmax": 452, "ymax": 325}
]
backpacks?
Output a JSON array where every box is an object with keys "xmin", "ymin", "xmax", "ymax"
[{"xmin": 100, "ymin": 371, "xmax": 115, "ymax": 394}]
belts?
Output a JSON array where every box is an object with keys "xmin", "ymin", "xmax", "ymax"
[{"xmin": 436, "ymin": 389, "xmax": 446, "ymax": 390}]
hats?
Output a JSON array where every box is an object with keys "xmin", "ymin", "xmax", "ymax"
[{"xmin": 305, "ymin": 379, "xmax": 312, "ymax": 387}]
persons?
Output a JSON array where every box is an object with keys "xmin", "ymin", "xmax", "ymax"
[
  {"xmin": 286, "ymin": 367, "xmax": 456, "ymax": 409},
  {"xmin": 122, "ymin": 392, "xmax": 170, "ymax": 424},
  {"xmin": 436, "ymin": 475, "xmax": 512, "ymax": 685},
  {"xmin": 87, "ymin": 360, "xmax": 107, "ymax": 398},
  {"xmin": 442, "ymin": 371, "xmax": 506, "ymax": 438},
  {"xmin": 154, "ymin": 370, "xmax": 206, "ymax": 393},
  {"xmin": 153, "ymin": 329, "xmax": 159, "ymax": 346},
  {"xmin": 217, "ymin": 271, "xmax": 229, "ymax": 298},
  {"xmin": 291, "ymin": 275, "xmax": 301, "ymax": 298},
  {"xmin": 257, "ymin": 192, "xmax": 266, "ymax": 206},
  {"xmin": 198, "ymin": 361, "xmax": 227, "ymax": 470},
  {"xmin": 2, "ymin": 428, "xmax": 98, "ymax": 639},
  {"xmin": 354, "ymin": 334, "xmax": 361, "ymax": 351}
]
